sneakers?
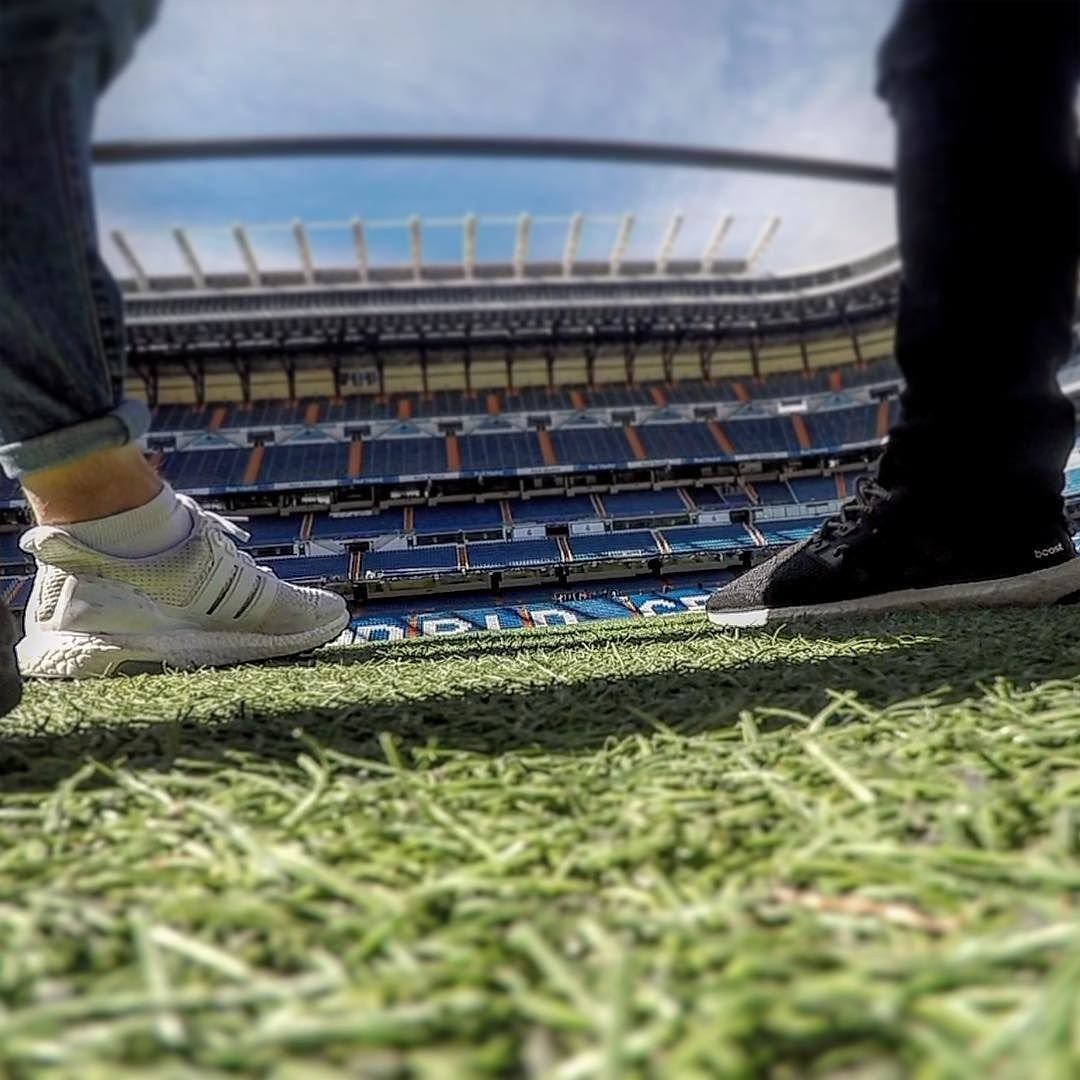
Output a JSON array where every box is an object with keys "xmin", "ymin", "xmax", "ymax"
[
  {"xmin": 15, "ymin": 492, "xmax": 349, "ymax": 679},
  {"xmin": 706, "ymin": 469, "xmax": 1080, "ymax": 628}
]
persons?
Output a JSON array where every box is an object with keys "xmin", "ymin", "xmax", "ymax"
[
  {"xmin": 0, "ymin": 0, "xmax": 351, "ymax": 681},
  {"xmin": 703, "ymin": 0, "xmax": 1080, "ymax": 625}
]
